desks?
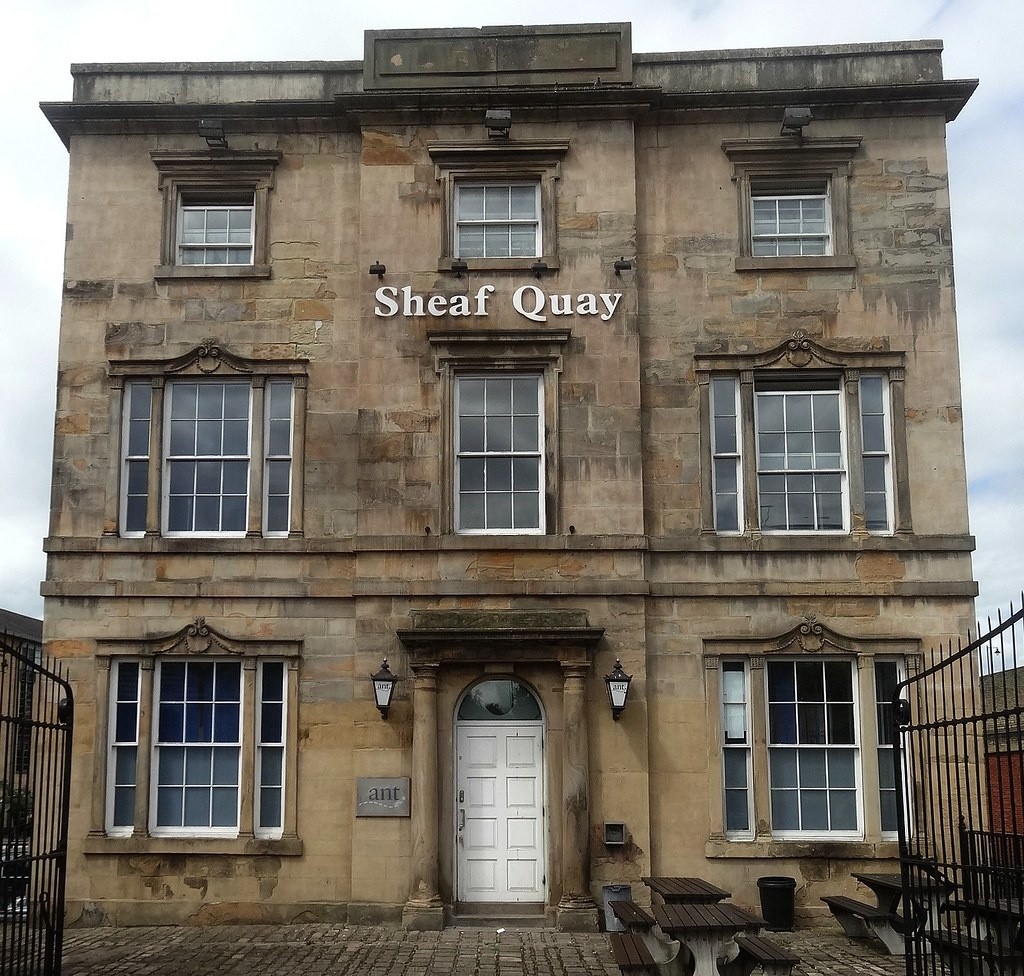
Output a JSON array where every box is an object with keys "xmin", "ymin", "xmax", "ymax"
[
  {"xmin": 923, "ymin": 898, "xmax": 1024, "ymax": 976},
  {"xmin": 618, "ymin": 904, "xmax": 794, "ymax": 976},
  {"xmin": 829, "ymin": 873, "xmax": 989, "ymax": 955},
  {"xmin": 617, "ymin": 878, "xmax": 759, "ymax": 963}
]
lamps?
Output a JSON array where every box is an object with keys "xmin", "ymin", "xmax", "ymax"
[
  {"xmin": 531, "ymin": 260, "xmax": 548, "ymax": 278},
  {"xmin": 197, "ymin": 120, "xmax": 230, "ymax": 149},
  {"xmin": 369, "ymin": 261, "xmax": 386, "ymax": 279},
  {"xmin": 613, "ymin": 257, "xmax": 632, "ymax": 275},
  {"xmin": 370, "ymin": 656, "xmax": 399, "ymax": 721},
  {"xmin": 485, "ymin": 109, "xmax": 513, "ymax": 139},
  {"xmin": 451, "ymin": 258, "xmax": 468, "ymax": 278},
  {"xmin": 603, "ymin": 658, "xmax": 633, "ymax": 723},
  {"xmin": 778, "ymin": 108, "xmax": 812, "ymax": 135}
]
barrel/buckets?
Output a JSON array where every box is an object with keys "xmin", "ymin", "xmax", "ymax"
[{"xmin": 755, "ymin": 876, "xmax": 797, "ymax": 930}]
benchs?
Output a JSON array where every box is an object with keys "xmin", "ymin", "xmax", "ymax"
[
  {"xmin": 919, "ymin": 929, "xmax": 1018, "ymax": 960},
  {"xmin": 610, "ymin": 933, "xmax": 656, "ymax": 968},
  {"xmin": 820, "ymin": 896, "xmax": 893, "ymax": 921},
  {"xmin": 735, "ymin": 935, "xmax": 801, "ymax": 963},
  {"xmin": 608, "ymin": 900, "xmax": 656, "ymax": 926},
  {"xmin": 942, "ymin": 898, "xmax": 966, "ymax": 910}
]
